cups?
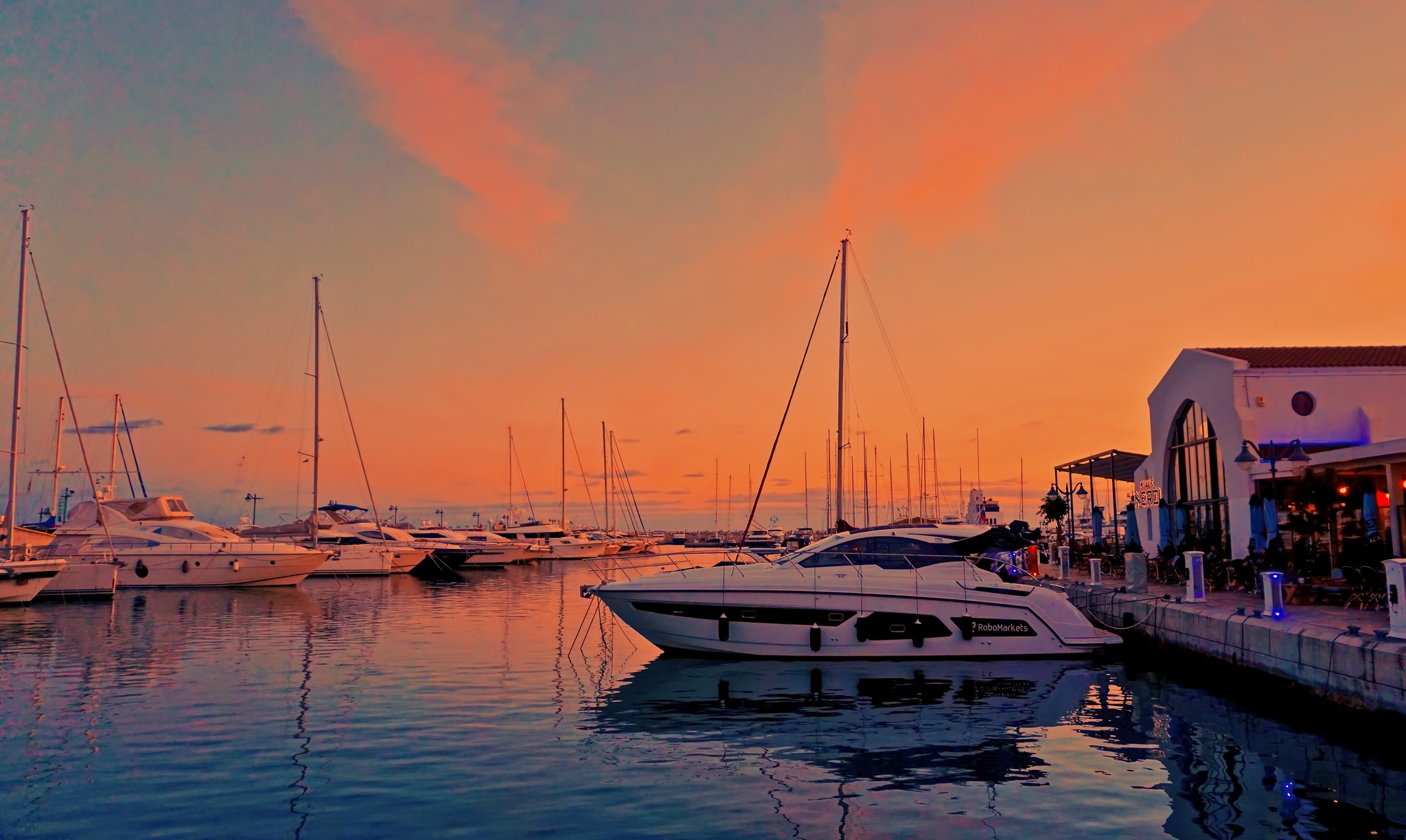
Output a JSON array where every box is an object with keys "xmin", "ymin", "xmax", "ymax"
[
  {"xmin": 1298, "ymin": 578, "xmax": 1305, "ymax": 584},
  {"xmin": 1223, "ymin": 559, "xmax": 1227, "ymax": 562},
  {"xmin": 1257, "ymin": 560, "xmax": 1261, "ymax": 563},
  {"xmin": 1250, "ymin": 560, "xmax": 1253, "ymax": 563},
  {"xmin": 1102, "ymin": 553, "xmax": 1106, "ymax": 555}
]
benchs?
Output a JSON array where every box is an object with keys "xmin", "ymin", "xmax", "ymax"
[{"xmin": 1282, "ymin": 584, "xmax": 1352, "ymax": 605}]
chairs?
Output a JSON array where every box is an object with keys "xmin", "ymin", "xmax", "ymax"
[
  {"xmin": 1071, "ymin": 552, "xmax": 1262, "ymax": 598},
  {"xmin": 1342, "ymin": 566, "xmax": 1389, "ymax": 612}
]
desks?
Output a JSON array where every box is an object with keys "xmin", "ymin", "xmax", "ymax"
[
  {"xmin": 1104, "ymin": 555, "xmax": 1115, "ymax": 576},
  {"xmin": 1218, "ymin": 561, "xmax": 1232, "ymax": 585},
  {"xmin": 1083, "ymin": 554, "xmax": 1094, "ymax": 573},
  {"xmin": 1306, "ymin": 576, "xmax": 1352, "ymax": 605},
  {"xmin": 1148, "ymin": 557, "xmax": 1162, "ymax": 580}
]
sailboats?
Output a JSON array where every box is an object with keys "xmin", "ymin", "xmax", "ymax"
[
  {"xmin": 578, "ymin": 226, "xmax": 1125, "ymax": 663},
  {"xmin": 1, "ymin": 208, "xmax": 437, "ymax": 610},
  {"xmin": 484, "ymin": 397, "xmax": 667, "ymax": 560}
]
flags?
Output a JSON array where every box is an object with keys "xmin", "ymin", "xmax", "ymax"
[{"xmin": 980, "ymin": 506, "xmax": 986, "ymax": 525}]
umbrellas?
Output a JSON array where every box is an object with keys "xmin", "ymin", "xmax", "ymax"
[
  {"xmin": 1125, "ymin": 504, "xmax": 1142, "ymax": 554},
  {"xmin": 1249, "ymin": 489, "xmax": 1278, "ymax": 551},
  {"xmin": 1091, "ymin": 505, "xmax": 1102, "ymax": 545},
  {"xmin": 1362, "ymin": 488, "xmax": 1378, "ymax": 538},
  {"xmin": 1157, "ymin": 497, "xmax": 1186, "ymax": 551}
]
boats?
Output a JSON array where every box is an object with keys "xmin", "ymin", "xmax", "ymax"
[{"xmin": 391, "ymin": 527, "xmax": 551, "ymax": 574}]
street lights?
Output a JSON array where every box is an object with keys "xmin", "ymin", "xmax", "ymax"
[{"xmin": 1046, "ymin": 481, "xmax": 1089, "ymax": 548}]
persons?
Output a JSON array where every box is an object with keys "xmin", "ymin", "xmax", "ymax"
[
  {"xmin": 1168, "ymin": 538, "xmax": 1345, "ymax": 596},
  {"xmin": 1374, "ymin": 556, "xmax": 1400, "ymax": 609}
]
